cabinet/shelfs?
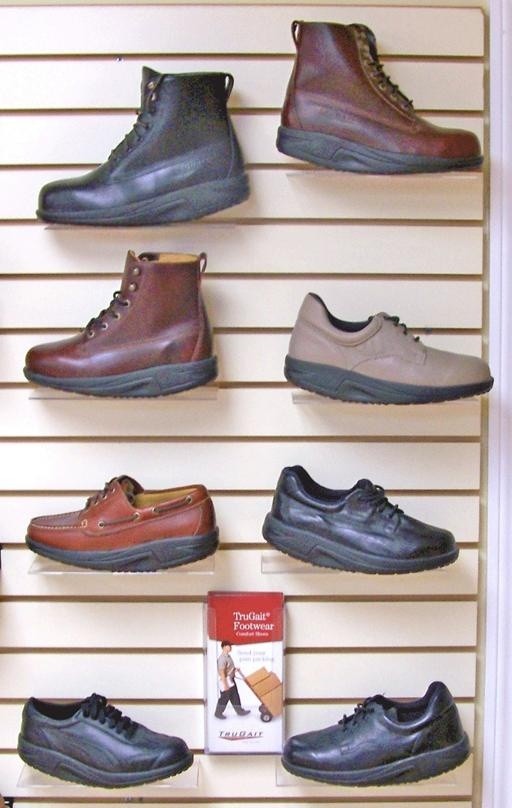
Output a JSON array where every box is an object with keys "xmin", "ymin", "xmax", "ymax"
[{"xmin": 0, "ymin": 163, "xmax": 493, "ymax": 808}]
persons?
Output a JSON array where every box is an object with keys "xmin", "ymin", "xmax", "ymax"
[{"xmin": 214, "ymin": 639, "xmax": 250, "ymax": 720}]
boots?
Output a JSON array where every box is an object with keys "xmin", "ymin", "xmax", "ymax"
[
  {"xmin": 23, "ymin": 250, "xmax": 217, "ymax": 398},
  {"xmin": 276, "ymin": 20, "xmax": 485, "ymax": 174},
  {"xmin": 36, "ymin": 66, "xmax": 250, "ymax": 225}
]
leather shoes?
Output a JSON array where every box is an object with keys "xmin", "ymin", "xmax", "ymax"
[
  {"xmin": 27, "ymin": 475, "xmax": 219, "ymax": 572},
  {"xmin": 17, "ymin": 693, "xmax": 193, "ymax": 789},
  {"xmin": 285, "ymin": 293, "xmax": 494, "ymax": 402},
  {"xmin": 262, "ymin": 466, "xmax": 460, "ymax": 575},
  {"xmin": 282, "ymin": 681, "xmax": 471, "ymax": 787}
]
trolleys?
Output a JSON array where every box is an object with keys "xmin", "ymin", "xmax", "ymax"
[{"xmin": 232, "ymin": 662, "xmax": 284, "ymax": 723}]
